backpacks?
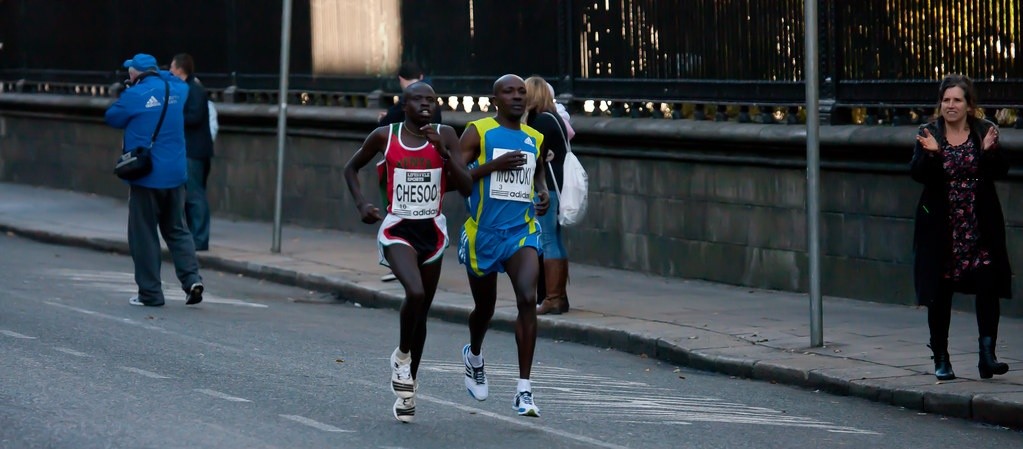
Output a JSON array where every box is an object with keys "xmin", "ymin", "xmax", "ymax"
[{"xmin": 541, "ymin": 112, "xmax": 588, "ymax": 227}]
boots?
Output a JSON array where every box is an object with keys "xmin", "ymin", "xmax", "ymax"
[
  {"xmin": 978, "ymin": 336, "xmax": 1009, "ymax": 378},
  {"xmin": 927, "ymin": 337, "xmax": 955, "ymax": 379},
  {"xmin": 535, "ymin": 255, "xmax": 570, "ymax": 315}
]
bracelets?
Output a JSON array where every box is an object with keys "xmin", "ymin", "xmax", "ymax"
[{"xmin": 439, "ymin": 150, "xmax": 451, "ymax": 160}]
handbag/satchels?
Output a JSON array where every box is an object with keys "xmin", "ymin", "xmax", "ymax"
[{"xmin": 113, "ymin": 145, "xmax": 153, "ymax": 180}]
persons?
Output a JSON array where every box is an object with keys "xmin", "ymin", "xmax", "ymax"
[
  {"xmin": 378, "ymin": 61, "xmax": 442, "ymax": 280},
  {"xmin": 908, "ymin": 75, "xmax": 1012, "ymax": 380},
  {"xmin": 459, "ymin": 74, "xmax": 551, "ymax": 417},
  {"xmin": 344, "ymin": 82, "xmax": 474, "ymax": 423},
  {"xmin": 520, "ymin": 76, "xmax": 568, "ymax": 314},
  {"xmin": 170, "ymin": 53, "xmax": 219, "ymax": 250},
  {"xmin": 104, "ymin": 53, "xmax": 204, "ymax": 306}
]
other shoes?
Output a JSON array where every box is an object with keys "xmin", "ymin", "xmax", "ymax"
[{"xmin": 381, "ymin": 270, "xmax": 398, "ymax": 281}]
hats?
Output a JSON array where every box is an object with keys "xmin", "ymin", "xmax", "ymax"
[{"xmin": 123, "ymin": 54, "xmax": 158, "ymax": 71}]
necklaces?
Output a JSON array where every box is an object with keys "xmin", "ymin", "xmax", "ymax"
[{"xmin": 402, "ymin": 120, "xmax": 424, "ymax": 137}]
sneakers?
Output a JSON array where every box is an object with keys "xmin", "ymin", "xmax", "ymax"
[
  {"xmin": 393, "ymin": 381, "xmax": 418, "ymax": 423},
  {"xmin": 186, "ymin": 282, "xmax": 204, "ymax": 304},
  {"xmin": 391, "ymin": 346, "xmax": 415, "ymax": 399},
  {"xmin": 462, "ymin": 344, "xmax": 488, "ymax": 401},
  {"xmin": 129, "ymin": 295, "xmax": 144, "ymax": 305},
  {"xmin": 512, "ymin": 390, "xmax": 541, "ymax": 417}
]
